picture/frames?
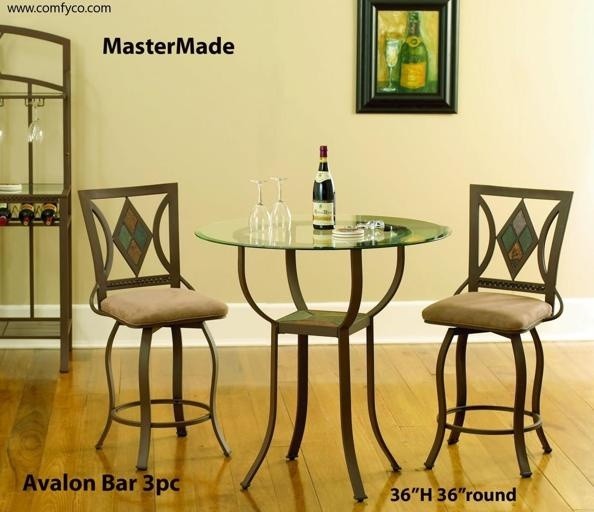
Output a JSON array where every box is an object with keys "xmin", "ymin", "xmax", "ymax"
[{"xmin": 356, "ymin": 0, "xmax": 457, "ymax": 114}]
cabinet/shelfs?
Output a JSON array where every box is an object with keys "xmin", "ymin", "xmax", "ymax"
[{"xmin": 0, "ymin": 24, "xmax": 74, "ymax": 373}]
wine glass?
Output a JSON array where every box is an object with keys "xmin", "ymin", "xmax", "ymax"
[
  {"xmin": 381, "ymin": 33, "xmax": 401, "ymax": 93},
  {"xmin": 249, "ymin": 176, "xmax": 291, "ymax": 236},
  {"xmin": 26, "ymin": 101, "xmax": 43, "ymax": 144}
]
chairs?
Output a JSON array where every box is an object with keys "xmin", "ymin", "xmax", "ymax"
[
  {"xmin": 421, "ymin": 183, "xmax": 575, "ymax": 479},
  {"xmin": 76, "ymin": 183, "xmax": 231, "ymax": 470}
]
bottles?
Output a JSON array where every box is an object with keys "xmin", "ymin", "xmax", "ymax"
[
  {"xmin": 400, "ymin": 12, "xmax": 429, "ymax": 92},
  {"xmin": 1, "ymin": 201, "xmax": 59, "ymax": 226},
  {"xmin": 310, "ymin": 143, "xmax": 335, "ymax": 230}
]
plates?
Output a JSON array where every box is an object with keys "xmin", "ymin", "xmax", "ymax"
[
  {"xmin": 332, "ymin": 228, "xmax": 365, "ymax": 238},
  {"xmin": 1, "ymin": 183, "xmax": 23, "ymax": 190}
]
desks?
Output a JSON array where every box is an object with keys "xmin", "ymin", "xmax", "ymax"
[{"xmin": 196, "ymin": 213, "xmax": 451, "ymax": 501}]
